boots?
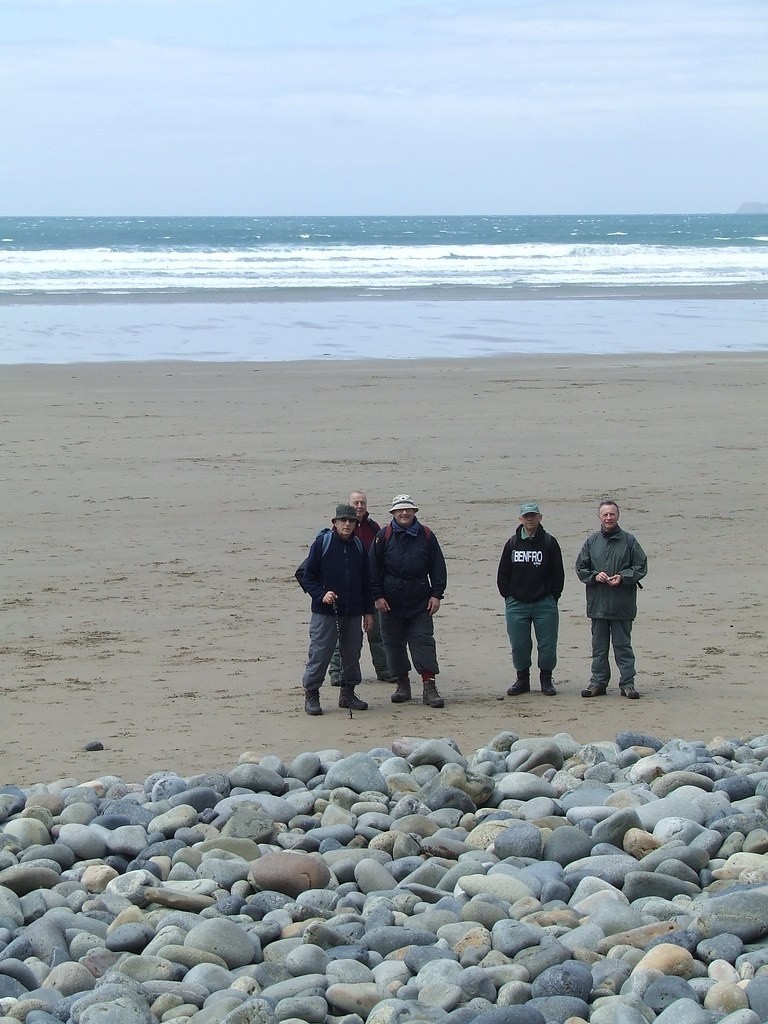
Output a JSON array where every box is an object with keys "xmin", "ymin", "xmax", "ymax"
[
  {"xmin": 392, "ymin": 677, "xmax": 411, "ymax": 703},
  {"xmin": 338, "ymin": 684, "xmax": 368, "ymax": 709},
  {"xmin": 507, "ymin": 668, "xmax": 530, "ymax": 695},
  {"xmin": 305, "ymin": 689, "xmax": 322, "ymax": 715},
  {"xmin": 422, "ymin": 678, "xmax": 444, "ymax": 707},
  {"xmin": 539, "ymin": 670, "xmax": 556, "ymax": 695}
]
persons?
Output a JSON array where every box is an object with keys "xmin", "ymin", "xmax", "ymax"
[
  {"xmin": 497, "ymin": 502, "xmax": 565, "ymax": 696},
  {"xmin": 575, "ymin": 501, "xmax": 648, "ymax": 699},
  {"xmin": 302, "ymin": 491, "xmax": 447, "ymax": 715}
]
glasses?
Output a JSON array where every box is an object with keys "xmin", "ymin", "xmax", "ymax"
[{"xmin": 337, "ymin": 518, "xmax": 356, "ymax": 523}]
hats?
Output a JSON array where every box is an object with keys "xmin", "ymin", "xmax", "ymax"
[
  {"xmin": 520, "ymin": 503, "xmax": 540, "ymax": 516},
  {"xmin": 388, "ymin": 494, "xmax": 419, "ymax": 514},
  {"xmin": 332, "ymin": 505, "xmax": 359, "ymax": 526}
]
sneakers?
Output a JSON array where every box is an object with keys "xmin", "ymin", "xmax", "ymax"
[
  {"xmin": 378, "ymin": 672, "xmax": 398, "ymax": 683},
  {"xmin": 330, "ymin": 674, "xmax": 341, "ymax": 686},
  {"xmin": 581, "ymin": 684, "xmax": 607, "ymax": 697},
  {"xmin": 622, "ymin": 686, "xmax": 639, "ymax": 699}
]
